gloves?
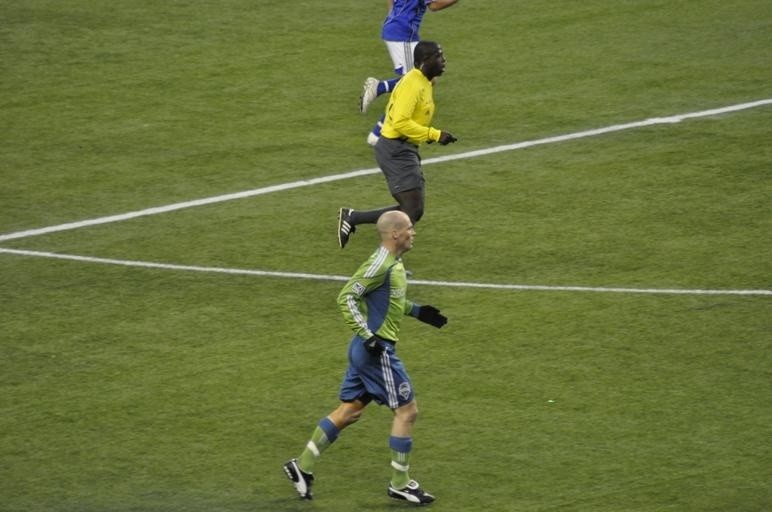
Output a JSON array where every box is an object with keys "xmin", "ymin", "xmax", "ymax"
[
  {"xmin": 363, "ymin": 335, "xmax": 388, "ymax": 359},
  {"xmin": 419, "ymin": 304, "xmax": 448, "ymax": 329}
]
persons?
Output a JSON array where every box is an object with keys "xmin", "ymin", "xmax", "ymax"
[
  {"xmin": 358, "ymin": 0, "xmax": 459, "ymax": 113},
  {"xmin": 337, "ymin": 41, "xmax": 457, "ymax": 248},
  {"xmin": 283, "ymin": 211, "xmax": 447, "ymax": 506}
]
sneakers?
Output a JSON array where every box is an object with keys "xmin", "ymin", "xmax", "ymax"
[
  {"xmin": 389, "ymin": 480, "xmax": 436, "ymax": 505},
  {"xmin": 359, "ymin": 76, "xmax": 381, "ymax": 145},
  {"xmin": 282, "ymin": 458, "xmax": 315, "ymax": 503},
  {"xmin": 338, "ymin": 207, "xmax": 354, "ymax": 249}
]
